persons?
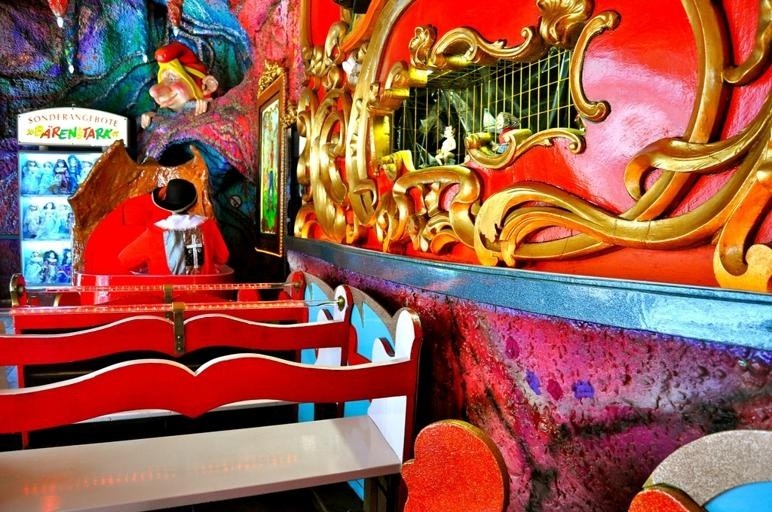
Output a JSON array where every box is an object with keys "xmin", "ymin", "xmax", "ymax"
[
  {"xmin": 117, "ymin": 178, "xmax": 230, "ymax": 276},
  {"xmin": 21, "ymin": 155, "xmax": 93, "ymax": 287}
]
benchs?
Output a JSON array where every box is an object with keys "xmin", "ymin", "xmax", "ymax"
[
  {"xmin": 8, "ymin": 271, "xmax": 306, "ymax": 309},
  {"xmin": 0, "ymin": 284, "xmax": 350, "ymax": 450},
  {"xmin": 0, "ymin": 309, "xmax": 422, "ymax": 512}
]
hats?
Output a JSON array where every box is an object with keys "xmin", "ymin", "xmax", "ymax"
[{"xmin": 152, "ymin": 178, "xmax": 197, "ymax": 214}]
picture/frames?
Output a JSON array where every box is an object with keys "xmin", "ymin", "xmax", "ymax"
[{"xmin": 254, "ymin": 55, "xmax": 289, "ymax": 260}]
